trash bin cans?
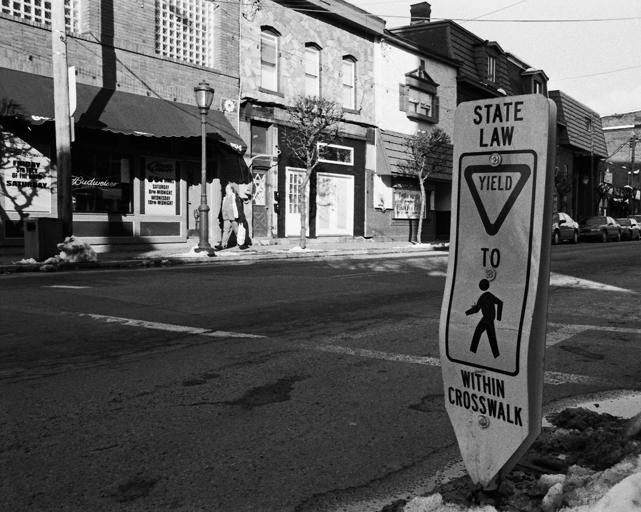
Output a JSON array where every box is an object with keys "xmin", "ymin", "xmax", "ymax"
[{"xmin": 23, "ymin": 217, "xmax": 63, "ymax": 262}]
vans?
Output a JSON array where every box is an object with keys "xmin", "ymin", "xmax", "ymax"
[{"xmin": 627, "ymin": 214, "xmax": 640, "ymax": 230}]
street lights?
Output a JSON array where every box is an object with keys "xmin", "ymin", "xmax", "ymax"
[{"xmin": 191, "ymin": 79, "xmax": 216, "ymax": 257}]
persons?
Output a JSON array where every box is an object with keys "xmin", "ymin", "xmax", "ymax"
[{"xmin": 215, "ymin": 183, "xmax": 247, "ymax": 249}]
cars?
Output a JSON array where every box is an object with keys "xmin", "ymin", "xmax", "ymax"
[
  {"xmin": 579, "ymin": 216, "xmax": 623, "ymax": 242},
  {"xmin": 551, "ymin": 212, "xmax": 581, "ymax": 244},
  {"xmin": 614, "ymin": 218, "xmax": 640, "ymax": 241}
]
